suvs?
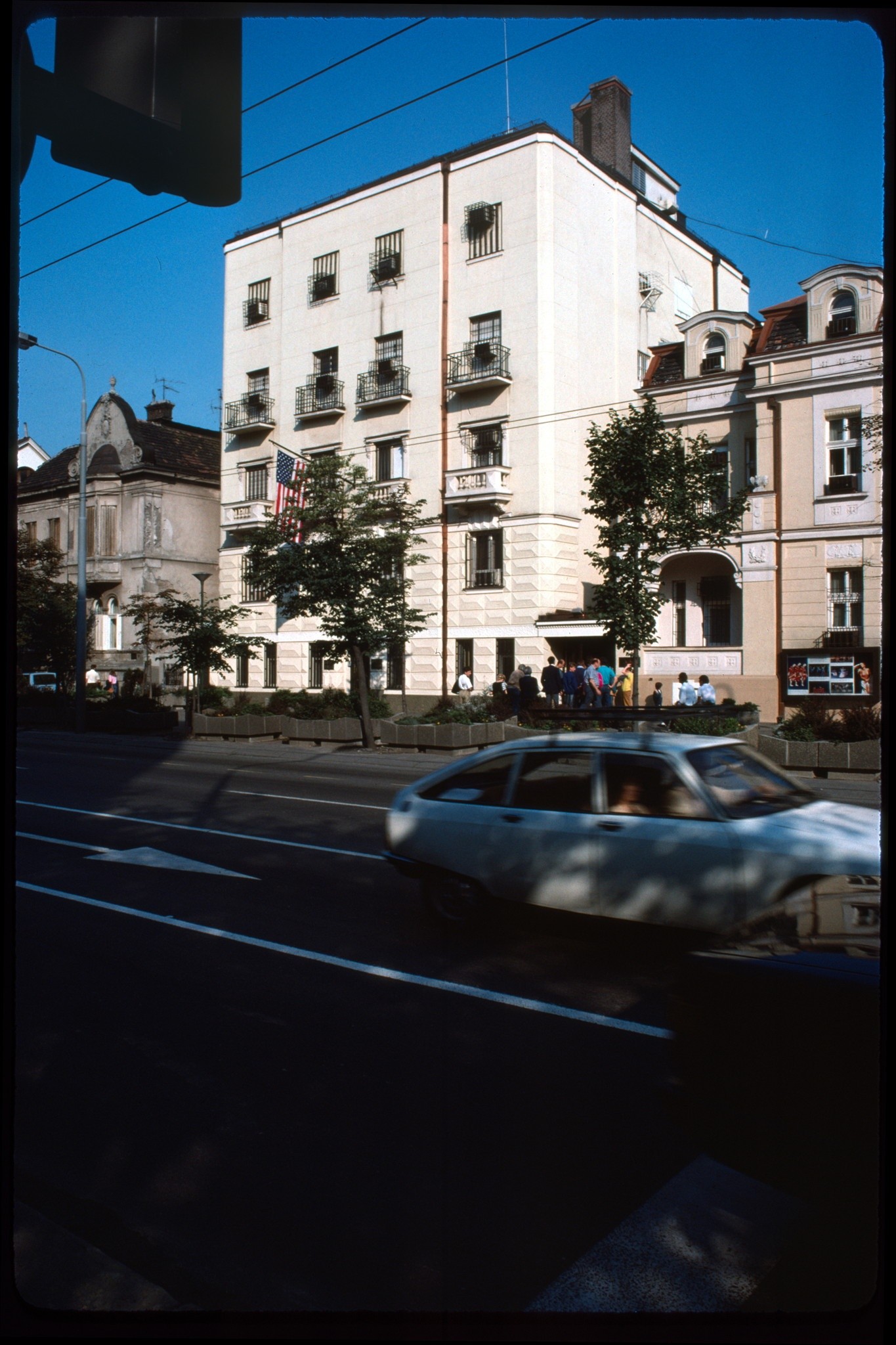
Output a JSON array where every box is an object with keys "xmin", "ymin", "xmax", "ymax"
[{"xmin": 20, "ymin": 670, "xmax": 65, "ymax": 693}]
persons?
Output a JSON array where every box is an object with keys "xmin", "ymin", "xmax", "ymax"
[
  {"xmin": 108, "ymin": 670, "xmax": 118, "ymax": 694},
  {"xmin": 839, "ymin": 667, "xmax": 846, "ymax": 678},
  {"xmin": 857, "ymin": 663, "xmax": 870, "ymax": 693},
  {"xmin": 810, "ymin": 665, "xmax": 825, "ymax": 676},
  {"xmin": 488, "ymin": 674, "xmax": 508, "ymax": 721},
  {"xmin": 610, "ymin": 775, "xmax": 650, "ymax": 814},
  {"xmin": 556, "ymin": 658, "xmax": 633, "ymax": 728},
  {"xmin": 458, "ymin": 667, "xmax": 473, "ymax": 705},
  {"xmin": 813, "ymin": 686, "xmax": 824, "ymax": 693},
  {"xmin": 519, "ymin": 667, "xmax": 540, "ymax": 707},
  {"xmin": 787, "ymin": 663, "xmax": 808, "ymax": 686},
  {"xmin": 697, "ymin": 675, "xmax": 716, "ymax": 705},
  {"xmin": 541, "ymin": 657, "xmax": 564, "ymax": 708},
  {"xmin": 653, "ymin": 683, "xmax": 663, "ymax": 710},
  {"xmin": 658, "ymin": 757, "xmax": 776, "ymax": 818},
  {"xmin": 656, "ymin": 673, "xmax": 697, "ymax": 731},
  {"xmin": 507, "ymin": 665, "xmax": 525, "ymax": 719},
  {"xmin": 832, "ymin": 683, "xmax": 848, "ymax": 693},
  {"xmin": 85, "ymin": 664, "xmax": 100, "ymax": 689}
]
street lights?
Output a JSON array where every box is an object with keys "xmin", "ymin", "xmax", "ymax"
[
  {"xmin": 191, "ymin": 571, "xmax": 213, "ymax": 713},
  {"xmin": 17, "ymin": 331, "xmax": 87, "ymax": 734}
]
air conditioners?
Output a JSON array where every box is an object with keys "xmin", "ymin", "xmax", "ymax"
[
  {"xmin": 246, "ymin": 393, "xmax": 266, "ymax": 407},
  {"xmin": 378, "ymin": 256, "xmax": 396, "ymax": 273},
  {"xmin": 478, "ymin": 431, "xmax": 499, "ymax": 446},
  {"xmin": 313, "ymin": 275, "xmax": 335, "ymax": 295},
  {"xmin": 468, "ymin": 206, "xmax": 494, "ymax": 225},
  {"xmin": 246, "ymin": 302, "xmax": 268, "ymax": 318},
  {"xmin": 377, "ymin": 359, "xmax": 399, "ymax": 374},
  {"xmin": 474, "ymin": 342, "xmax": 497, "ymax": 358},
  {"xmin": 315, "ymin": 374, "xmax": 335, "ymax": 390}
]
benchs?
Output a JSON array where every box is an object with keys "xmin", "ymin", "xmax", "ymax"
[{"xmin": 530, "ymin": 706, "xmax": 730, "ymax": 720}]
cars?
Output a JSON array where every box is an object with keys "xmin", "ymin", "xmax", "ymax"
[{"xmin": 386, "ymin": 711, "xmax": 881, "ymax": 943}]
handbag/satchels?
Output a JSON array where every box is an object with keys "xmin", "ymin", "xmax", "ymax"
[{"xmin": 451, "ymin": 680, "xmax": 461, "ymax": 694}]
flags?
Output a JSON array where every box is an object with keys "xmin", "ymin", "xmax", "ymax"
[{"xmin": 276, "ymin": 450, "xmax": 309, "ymax": 543}]
